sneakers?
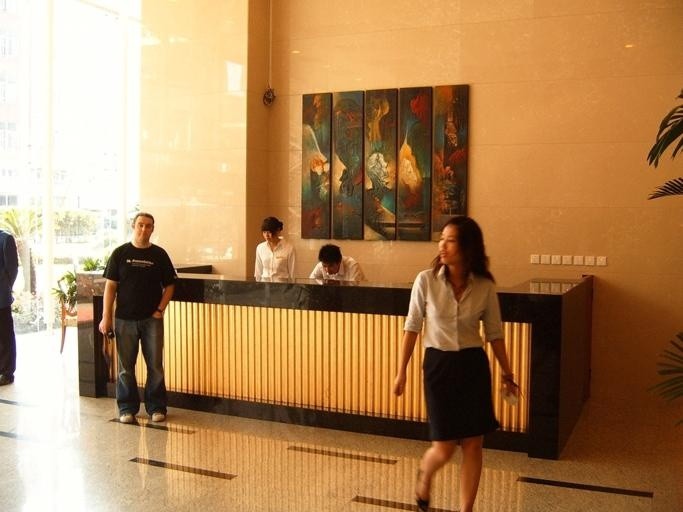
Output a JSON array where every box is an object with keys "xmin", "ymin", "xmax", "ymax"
[
  {"xmin": 152, "ymin": 413, "xmax": 165, "ymax": 422},
  {"xmin": 119, "ymin": 413, "xmax": 133, "ymax": 423}
]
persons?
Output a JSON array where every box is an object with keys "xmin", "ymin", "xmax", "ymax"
[
  {"xmin": 309, "ymin": 244, "xmax": 366, "ymax": 281},
  {"xmin": 394, "ymin": 216, "xmax": 518, "ymax": 512},
  {"xmin": 254, "ymin": 217, "xmax": 296, "ymax": 279},
  {"xmin": 98, "ymin": 213, "xmax": 179, "ymax": 424},
  {"xmin": 0, "ymin": 230, "xmax": 18, "ymax": 386}
]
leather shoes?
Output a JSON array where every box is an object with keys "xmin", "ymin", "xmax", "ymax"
[
  {"xmin": 0, "ymin": 375, "xmax": 14, "ymax": 386},
  {"xmin": 414, "ymin": 468, "xmax": 430, "ymax": 512}
]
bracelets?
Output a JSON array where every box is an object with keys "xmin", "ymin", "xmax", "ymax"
[
  {"xmin": 156, "ymin": 308, "xmax": 162, "ymax": 313},
  {"xmin": 501, "ymin": 373, "xmax": 514, "ymax": 382}
]
